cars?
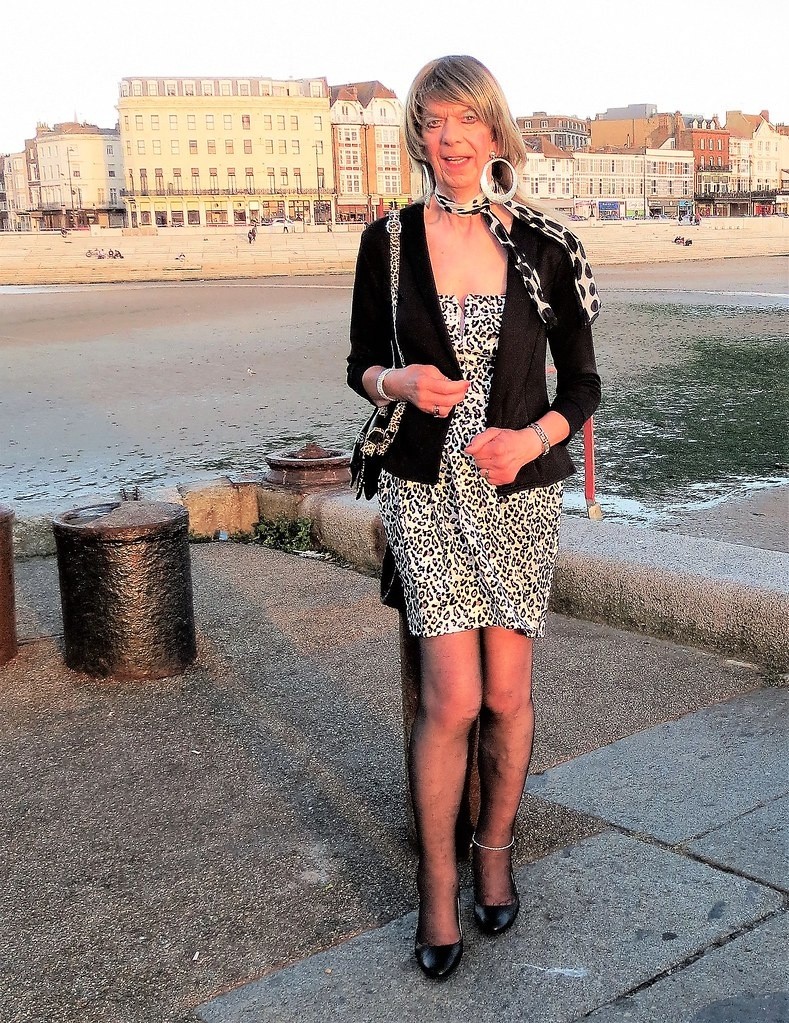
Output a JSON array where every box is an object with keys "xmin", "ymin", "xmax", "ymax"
[
  {"xmin": 262, "ymin": 218, "xmax": 294, "ymax": 227},
  {"xmin": 568, "ymin": 213, "xmax": 669, "ymax": 221},
  {"xmin": 778, "ymin": 212, "xmax": 788, "ymax": 218}
]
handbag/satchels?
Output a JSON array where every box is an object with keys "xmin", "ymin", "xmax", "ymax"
[{"xmin": 349, "ymin": 403, "xmax": 391, "ymax": 501}]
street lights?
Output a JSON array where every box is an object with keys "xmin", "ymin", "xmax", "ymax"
[{"xmin": 67, "ymin": 146, "xmax": 76, "ymax": 220}]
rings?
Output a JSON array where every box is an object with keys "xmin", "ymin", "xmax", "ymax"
[
  {"xmin": 485, "ymin": 468, "xmax": 490, "ymax": 478},
  {"xmin": 433, "ymin": 405, "xmax": 439, "ymax": 417}
]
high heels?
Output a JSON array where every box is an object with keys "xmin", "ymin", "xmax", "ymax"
[
  {"xmin": 474, "ymin": 859, "xmax": 519, "ymax": 934},
  {"xmin": 415, "ymin": 881, "xmax": 463, "ymax": 978}
]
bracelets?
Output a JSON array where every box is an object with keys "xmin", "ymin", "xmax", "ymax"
[
  {"xmin": 527, "ymin": 422, "xmax": 550, "ymax": 459},
  {"xmin": 376, "ymin": 369, "xmax": 403, "ymax": 401}
]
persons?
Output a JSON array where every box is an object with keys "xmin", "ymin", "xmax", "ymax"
[
  {"xmin": 62, "ymin": 228, "xmax": 67, "ymax": 238},
  {"xmin": 336, "ymin": 216, "xmax": 364, "ymax": 225},
  {"xmin": 171, "ymin": 222, "xmax": 183, "ymax": 227},
  {"xmin": 327, "ymin": 219, "xmax": 332, "ymax": 233},
  {"xmin": 678, "ymin": 212, "xmax": 702, "ymax": 226},
  {"xmin": 248, "ymin": 228, "xmax": 258, "ymax": 244},
  {"xmin": 86, "ymin": 248, "xmax": 124, "ymax": 259},
  {"xmin": 251, "ymin": 218, "xmax": 273, "ymax": 227},
  {"xmin": 282, "ymin": 218, "xmax": 289, "ymax": 234},
  {"xmin": 180, "ymin": 253, "xmax": 185, "ymax": 262},
  {"xmin": 675, "ymin": 236, "xmax": 692, "ymax": 246},
  {"xmin": 346, "ymin": 55, "xmax": 601, "ymax": 979}
]
